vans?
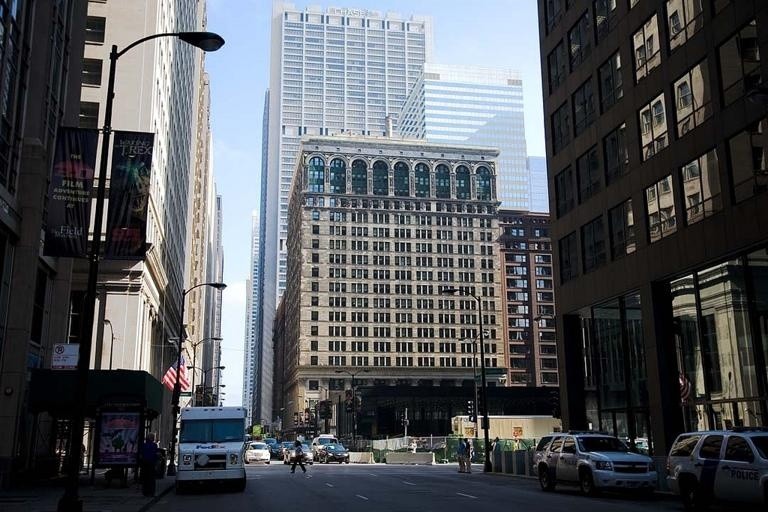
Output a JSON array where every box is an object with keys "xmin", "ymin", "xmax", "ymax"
[{"xmin": 174, "ymin": 405, "xmax": 248, "ymax": 494}]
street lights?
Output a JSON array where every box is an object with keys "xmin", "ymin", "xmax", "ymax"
[
  {"xmin": 165, "ymin": 335, "xmax": 227, "ymax": 406},
  {"xmin": 165, "ymin": 281, "xmax": 227, "ymax": 475},
  {"xmin": 76, "ymin": 30, "xmax": 226, "ymax": 371},
  {"xmin": 279, "ymin": 368, "xmax": 373, "ymax": 454},
  {"xmin": 101, "ymin": 318, "xmax": 115, "ymax": 369},
  {"xmin": 440, "ymin": 286, "xmax": 493, "ymax": 473}
]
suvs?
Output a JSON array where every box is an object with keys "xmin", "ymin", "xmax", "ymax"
[
  {"xmin": 665, "ymin": 429, "xmax": 767, "ymax": 511},
  {"xmin": 531, "ymin": 432, "xmax": 659, "ymax": 492}
]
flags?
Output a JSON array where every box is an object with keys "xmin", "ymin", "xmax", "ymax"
[
  {"xmin": 160, "ymin": 355, "xmax": 191, "ymax": 393},
  {"xmin": 678, "ymin": 372, "xmax": 692, "ymax": 406}
]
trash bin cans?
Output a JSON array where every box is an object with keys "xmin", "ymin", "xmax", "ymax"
[{"xmin": 493, "ymin": 438, "xmax": 533, "ymax": 476}]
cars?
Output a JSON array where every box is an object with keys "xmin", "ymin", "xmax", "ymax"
[{"xmin": 245, "ymin": 432, "xmax": 350, "ymax": 465}]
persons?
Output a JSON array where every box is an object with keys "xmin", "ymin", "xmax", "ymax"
[
  {"xmin": 140, "ymin": 433, "xmax": 158, "ymax": 497},
  {"xmin": 456, "ymin": 437, "xmax": 466, "ymax": 472},
  {"xmin": 407, "ymin": 438, "xmax": 418, "ymax": 454},
  {"xmin": 491, "ymin": 436, "xmax": 499, "ymax": 450},
  {"xmin": 462, "ymin": 438, "xmax": 472, "ymax": 473},
  {"xmin": 289, "ymin": 440, "xmax": 306, "ymax": 473}
]
